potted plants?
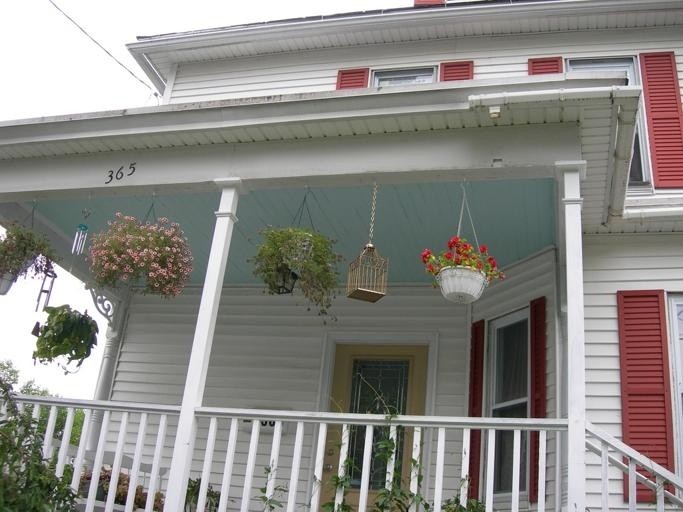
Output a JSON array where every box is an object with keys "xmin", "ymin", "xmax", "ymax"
[
  {"xmin": 243, "ymin": 223, "xmax": 341, "ymax": 326},
  {"xmin": 0, "ymin": 221, "xmax": 63, "ymax": 295},
  {"xmin": 80, "ymin": 470, "xmax": 110, "ymax": 501}
]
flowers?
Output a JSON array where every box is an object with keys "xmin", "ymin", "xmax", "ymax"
[
  {"xmin": 420, "ymin": 236, "xmax": 506, "ymax": 290},
  {"xmin": 89, "ymin": 211, "xmax": 194, "ymax": 298}
]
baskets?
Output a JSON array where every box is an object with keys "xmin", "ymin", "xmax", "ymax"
[
  {"xmin": 0, "ymin": 270, "xmax": 16, "ymax": 296},
  {"xmin": 20, "ymin": 247, "xmax": 38, "ymax": 269}
]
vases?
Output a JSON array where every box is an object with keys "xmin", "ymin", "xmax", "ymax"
[{"xmin": 125, "ymin": 270, "xmax": 151, "ymax": 295}]
